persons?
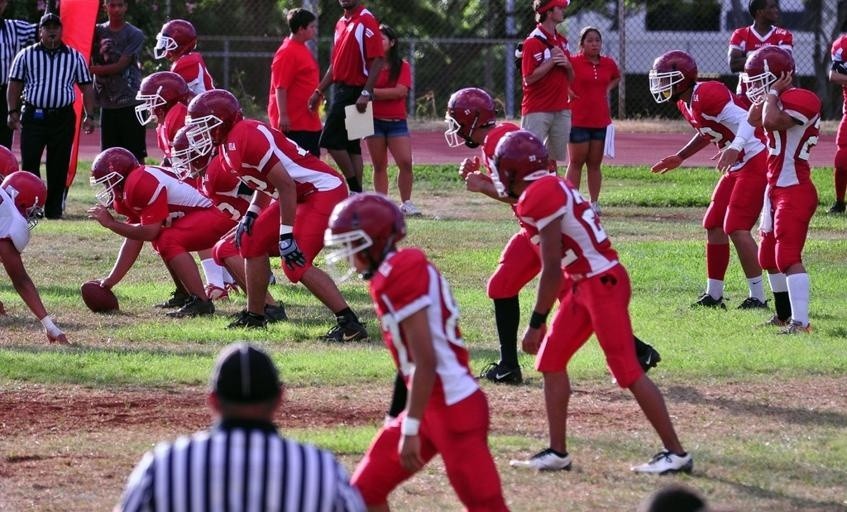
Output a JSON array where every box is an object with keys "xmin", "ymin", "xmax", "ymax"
[
  {"xmin": 726, "ymin": 2, "xmax": 793, "ymax": 105},
  {"xmin": 564, "ymin": 27, "xmax": 621, "ymax": 215},
  {"xmin": 90, "ymin": 1, "xmax": 148, "ymax": 210},
  {"xmin": 0, "ymin": 2, "xmax": 95, "ymax": 221},
  {"xmin": 327, "ymin": 193, "xmax": 509, "ymax": 510},
  {"xmin": 493, "ymin": 137, "xmax": 698, "ymax": 476},
  {"xmin": 184, "ymin": 90, "xmax": 370, "ymax": 344},
  {"xmin": 267, "ymin": 7, "xmax": 322, "ymax": 160},
  {"xmin": 82, "ymin": 20, "xmax": 289, "ymax": 324},
  {"xmin": 823, "ymin": 33, "xmax": 846, "ymax": 215},
  {"xmin": 743, "ymin": 46, "xmax": 820, "ymax": 336},
  {"xmin": 107, "ymin": 341, "xmax": 375, "ymax": 512},
  {"xmin": 445, "ymin": 87, "xmax": 661, "ymax": 384},
  {"xmin": 518, "ymin": 1, "xmax": 575, "ymax": 173},
  {"xmin": 362, "ymin": 24, "xmax": 421, "ymax": 217},
  {"xmin": 307, "ymin": 0, "xmax": 386, "ymax": 193},
  {"xmin": 647, "ymin": 51, "xmax": 769, "ymax": 312},
  {"xmin": 0, "ymin": 145, "xmax": 73, "ymax": 347}
]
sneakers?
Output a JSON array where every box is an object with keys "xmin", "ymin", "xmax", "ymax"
[
  {"xmin": 631, "ymin": 450, "xmax": 695, "ymax": 477},
  {"xmin": 633, "ymin": 339, "xmax": 661, "ymax": 373},
  {"xmin": 473, "ymin": 360, "xmax": 524, "ymax": 387},
  {"xmin": 399, "ymin": 200, "xmax": 422, "ymax": 217},
  {"xmin": 826, "ymin": 201, "xmax": 846, "ymax": 215},
  {"xmin": 154, "ymin": 290, "xmax": 215, "ymax": 318},
  {"xmin": 509, "ymin": 448, "xmax": 574, "ymax": 470},
  {"xmin": 590, "ymin": 202, "xmax": 602, "ymax": 216},
  {"xmin": 318, "ymin": 320, "xmax": 367, "ymax": 343},
  {"xmin": 688, "ymin": 295, "xmax": 812, "ymax": 338},
  {"xmin": 224, "ymin": 300, "xmax": 288, "ymax": 330}
]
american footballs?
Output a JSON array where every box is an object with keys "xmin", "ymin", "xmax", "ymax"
[{"xmin": 80, "ymin": 279, "xmax": 119, "ymax": 310}]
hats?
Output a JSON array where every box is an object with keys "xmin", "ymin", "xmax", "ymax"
[{"xmin": 533, "ymin": 0, "xmax": 571, "ymax": 13}]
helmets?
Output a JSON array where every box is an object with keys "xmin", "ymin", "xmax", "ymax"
[
  {"xmin": 738, "ymin": 46, "xmax": 795, "ymax": 105},
  {"xmin": 482, "ymin": 121, "xmax": 550, "ymax": 196},
  {"xmin": 89, "ymin": 146, "xmax": 139, "ymax": 205},
  {"xmin": 445, "ymin": 88, "xmax": 492, "ymax": 147},
  {"xmin": 170, "ymin": 88, "xmax": 241, "ymax": 181},
  {"xmin": 154, "ymin": 18, "xmax": 198, "ymax": 62},
  {"xmin": 0, "ymin": 144, "xmax": 47, "ymax": 228},
  {"xmin": 135, "ymin": 72, "xmax": 189, "ymax": 124},
  {"xmin": 647, "ymin": 49, "xmax": 699, "ymax": 104},
  {"xmin": 325, "ymin": 192, "xmax": 409, "ymax": 274}
]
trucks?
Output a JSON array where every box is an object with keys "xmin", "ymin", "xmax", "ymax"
[{"xmin": 567, "ymin": 0, "xmax": 840, "ymax": 113}]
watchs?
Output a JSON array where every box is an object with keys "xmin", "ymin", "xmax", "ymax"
[{"xmin": 361, "ymin": 89, "xmax": 370, "ymax": 96}]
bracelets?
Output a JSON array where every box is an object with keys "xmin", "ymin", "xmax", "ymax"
[
  {"xmin": 315, "ymin": 88, "xmax": 323, "ymax": 96},
  {"xmin": 542, "ymin": 306, "xmax": 550, "ymax": 323},
  {"xmin": 526, "ymin": 310, "xmax": 543, "ymax": 329},
  {"xmin": 400, "ymin": 416, "xmax": 420, "ymax": 437}
]
